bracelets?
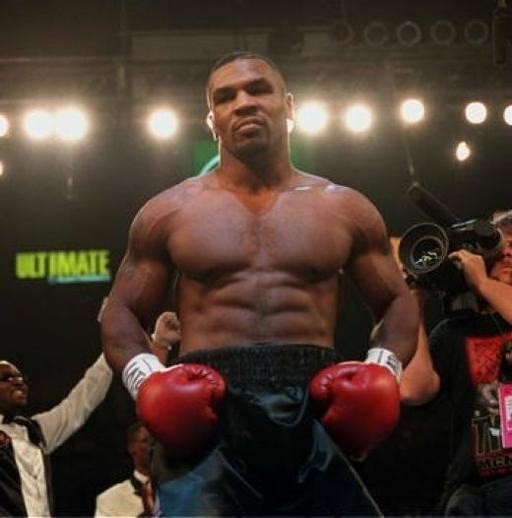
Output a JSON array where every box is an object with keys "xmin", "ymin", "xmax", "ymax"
[{"xmin": 150, "ymin": 334, "xmax": 173, "ymax": 352}]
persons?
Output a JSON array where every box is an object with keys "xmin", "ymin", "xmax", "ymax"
[
  {"xmin": 94, "ymin": 421, "xmax": 158, "ymax": 518},
  {"xmin": 150, "ymin": 312, "xmax": 184, "ymax": 366},
  {"xmin": 0, "ymin": 294, "xmax": 117, "ymax": 517},
  {"xmin": 95, "ymin": 49, "xmax": 422, "ymax": 518},
  {"xmin": 396, "ymin": 209, "xmax": 512, "ymax": 518}
]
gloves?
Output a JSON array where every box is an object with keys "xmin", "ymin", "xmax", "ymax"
[
  {"xmin": 309, "ymin": 348, "xmax": 403, "ymax": 456},
  {"xmin": 122, "ymin": 354, "xmax": 226, "ymax": 448}
]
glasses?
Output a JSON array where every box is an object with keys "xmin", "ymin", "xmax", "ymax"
[{"xmin": 0, "ymin": 373, "xmax": 25, "ymax": 382}]
